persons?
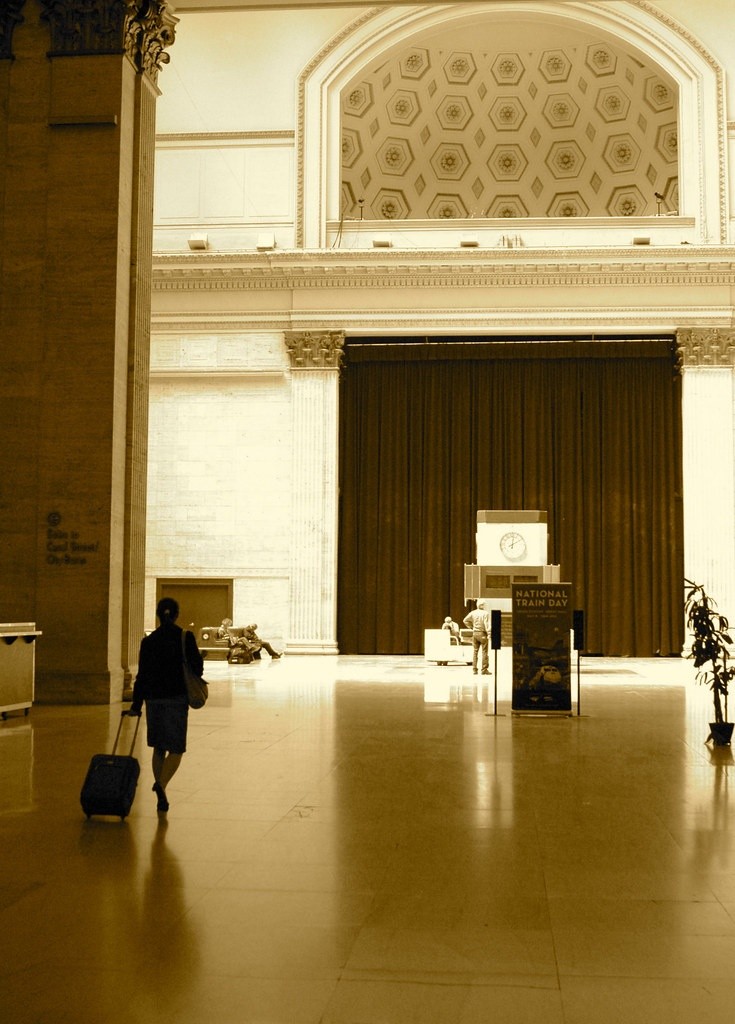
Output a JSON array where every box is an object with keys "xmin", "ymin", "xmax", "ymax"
[
  {"xmin": 242, "ymin": 624, "xmax": 283, "ymax": 659},
  {"xmin": 130, "ymin": 596, "xmax": 204, "ymax": 812},
  {"xmin": 442, "ymin": 616, "xmax": 461, "ymax": 645},
  {"xmin": 218, "ymin": 618, "xmax": 261, "ymax": 654},
  {"xmin": 463, "ymin": 599, "xmax": 492, "ymax": 675}
]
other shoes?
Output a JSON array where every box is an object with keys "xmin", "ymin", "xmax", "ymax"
[
  {"xmin": 272, "ymin": 653, "xmax": 283, "ymax": 658},
  {"xmin": 473, "ymin": 668, "xmax": 479, "ymax": 674},
  {"xmin": 482, "ymin": 669, "xmax": 492, "ymax": 675}
]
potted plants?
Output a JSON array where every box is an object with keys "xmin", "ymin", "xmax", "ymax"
[{"xmin": 681, "ymin": 578, "xmax": 735, "ymax": 745}]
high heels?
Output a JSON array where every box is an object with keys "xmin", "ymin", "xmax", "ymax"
[{"xmin": 152, "ymin": 781, "xmax": 169, "ymax": 813}]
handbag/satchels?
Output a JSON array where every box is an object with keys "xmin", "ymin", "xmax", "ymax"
[
  {"xmin": 184, "ymin": 674, "xmax": 209, "ymax": 709},
  {"xmin": 80, "ymin": 710, "xmax": 142, "ymax": 822}
]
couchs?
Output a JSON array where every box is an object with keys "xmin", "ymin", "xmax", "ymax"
[{"xmin": 195, "ymin": 627, "xmax": 243, "ymax": 661}]
